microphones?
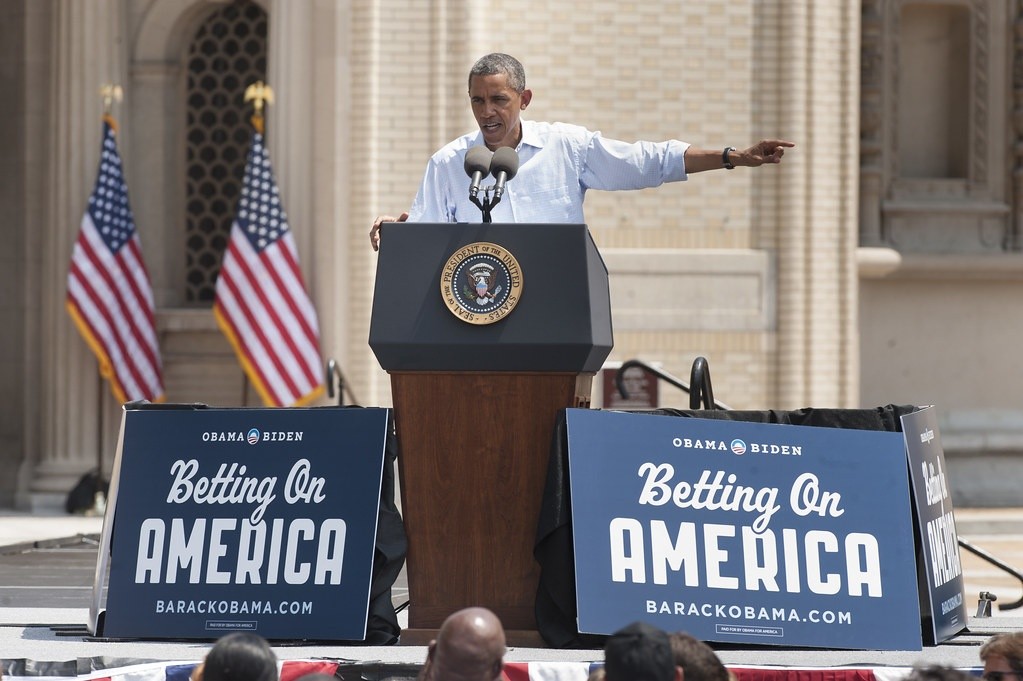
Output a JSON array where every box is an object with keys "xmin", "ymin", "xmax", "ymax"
[
  {"xmin": 464, "ymin": 146, "xmax": 491, "ymax": 198},
  {"xmin": 490, "ymin": 146, "xmax": 519, "ymax": 202}
]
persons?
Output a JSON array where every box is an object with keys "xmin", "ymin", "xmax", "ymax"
[
  {"xmin": 902, "ymin": 632, "xmax": 1023, "ymax": 681},
  {"xmin": 588, "ymin": 622, "xmax": 738, "ymax": 681},
  {"xmin": 369, "ymin": 53, "xmax": 795, "ymax": 251},
  {"xmin": 421, "ymin": 607, "xmax": 512, "ymax": 681},
  {"xmin": 188, "ymin": 632, "xmax": 341, "ymax": 681}
]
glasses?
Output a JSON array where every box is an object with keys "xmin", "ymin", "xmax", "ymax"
[{"xmin": 982, "ymin": 671, "xmax": 1023, "ymax": 681}]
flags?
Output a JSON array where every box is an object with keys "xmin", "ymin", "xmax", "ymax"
[
  {"xmin": 212, "ymin": 130, "xmax": 328, "ymax": 406},
  {"xmin": 67, "ymin": 112, "xmax": 166, "ymax": 406}
]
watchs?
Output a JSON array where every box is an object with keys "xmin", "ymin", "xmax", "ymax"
[{"xmin": 722, "ymin": 147, "xmax": 736, "ymax": 170}]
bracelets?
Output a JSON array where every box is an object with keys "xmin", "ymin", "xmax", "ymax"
[{"xmin": 189, "ymin": 677, "xmax": 192, "ymax": 681}]
